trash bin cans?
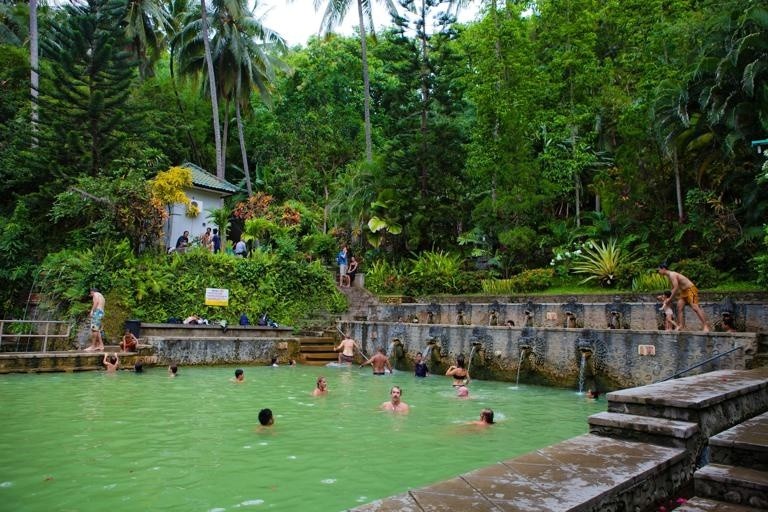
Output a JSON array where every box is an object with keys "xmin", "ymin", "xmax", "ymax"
[
  {"xmin": 125, "ymin": 319, "xmax": 141, "ymax": 339},
  {"xmin": 355, "ymin": 273, "xmax": 365, "ymax": 289}
]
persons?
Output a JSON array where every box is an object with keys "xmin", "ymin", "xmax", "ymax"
[
  {"xmin": 657, "ymin": 290, "xmax": 680, "ymax": 331},
  {"xmin": 207, "ymin": 227, "xmax": 220, "ymax": 253},
  {"xmin": 103, "ymin": 352, "xmax": 120, "ymax": 372},
  {"xmin": 201, "ymin": 232, "xmax": 210, "ymax": 245},
  {"xmin": 334, "ymin": 334, "xmax": 359, "ymax": 369},
  {"xmin": 312, "ymin": 376, "xmax": 328, "ymax": 396},
  {"xmin": 202, "ymin": 228, "xmax": 213, "ymax": 251},
  {"xmin": 270, "ymin": 357, "xmax": 280, "ymax": 367},
  {"xmin": 414, "ymin": 351, "xmax": 430, "ymax": 378},
  {"xmin": 461, "ymin": 406, "xmax": 494, "ymax": 430},
  {"xmin": 454, "ymin": 386, "xmax": 472, "ymax": 400},
  {"xmin": 338, "ymin": 246, "xmax": 348, "ymax": 286},
  {"xmin": 380, "ymin": 385, "xmax": 409, "ymax": 411},
  {"xmin": 256, "ymin": 408, "xmax": 276, "ymax": 433},
  {"xmin": 119, "ymin": 329, "xmax": 138, "ymax": 352},
  {"xmin": 721, "ymin": 318, "xmax": 738, "ymax": 332},
  {"xmin": 656, "ymin": 261, "xmax": 710, "ymax": 332},
  {"xmin": 232, "ymin": 368, "xmax": 245, "ymax": 382},
  {"xmin": 446, "ymin": 355, "xmax": 471, "ymax": 387},
  {"xmin": 134, "ymin": 361, "xmax": 143, "ymax": 373},
  {"xmin": 344, "ymin": 256, "xmax": 359, "ymax": 287},
  {"xmin": 168, "ymin": 364, "xmax": 177, "ymax": 377},
  {"xmin": 175, "ymin": 230, "xmax": 193, "ymax": 249},
  {"xmin": 586, "ymin": 387, "xmax": 598, "ymax": 400},
  {"xmin": 289, "ymin": 359, "xmax": 297, "ymax": 367},
  {"xmin": 359, "ymin": 346, "xmax": 393, "ymax": 376},
  {"xmin": 234, "ymin": 236, "xmax": 247, "ymax": 258},
  {"xmin": 83, "ymin": 288, "xmax": 106, "ymax": 351}
]
